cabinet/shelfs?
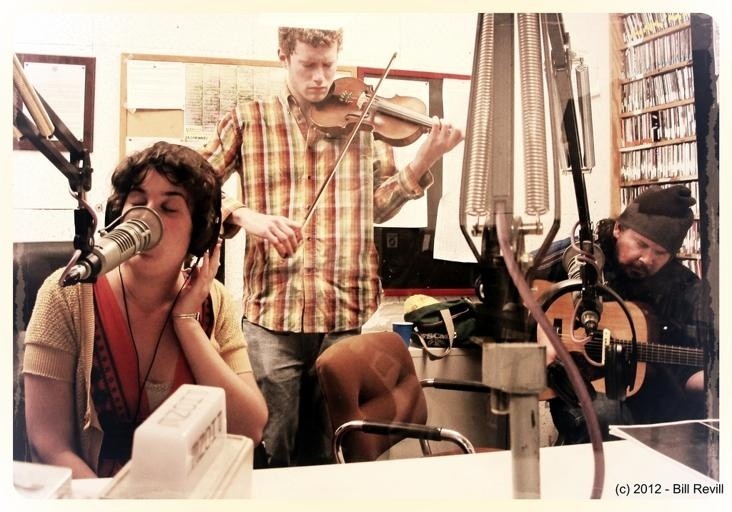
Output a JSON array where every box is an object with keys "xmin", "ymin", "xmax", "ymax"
[
  {"xmin": 380, "ymin": 344, "xmax": 502, "ymax": 458},
  {"xmin": 608, "ymin": 12, "xmax": 716, "ymax": 280}
]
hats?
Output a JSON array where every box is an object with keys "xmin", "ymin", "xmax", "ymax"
[{"xmin": 618, "ymin": 185, "xmax": 696, "ymax": 252}]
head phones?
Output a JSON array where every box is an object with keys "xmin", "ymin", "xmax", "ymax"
[{"xmin": 105, "ymin": 145, "xmax": 221, "ymax": 256}]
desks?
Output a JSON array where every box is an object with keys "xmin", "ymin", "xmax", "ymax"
[{"xmin": 45, "ymin": 438, "xmax": 710, "ymax": 499}]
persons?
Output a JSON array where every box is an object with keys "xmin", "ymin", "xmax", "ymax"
[
  {"xmin": 196, "ymin": 26, "xmax": 465, "ymax": 467},
  {"xmin": 478, "ymin": 171, "xmax": 708, "ymax": 446},
  {"xmin": 14, "ymin": 136, "xmax": 271, "ymax": 482}
]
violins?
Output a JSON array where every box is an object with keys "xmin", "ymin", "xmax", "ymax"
[{"xmin": 309, "ymin": 77, "xmax": 454, "ymax": 147}]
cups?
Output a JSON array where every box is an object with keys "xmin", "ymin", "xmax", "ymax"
[{"xmin": 391, "ymin": 321, "xmax": 414, "ymax": 348}]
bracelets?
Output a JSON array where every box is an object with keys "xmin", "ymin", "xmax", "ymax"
[{"xmin": 173, "ymin": 310, "xmax": 202, "ymax": 323}]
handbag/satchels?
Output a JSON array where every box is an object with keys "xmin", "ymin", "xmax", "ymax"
[{"xmin": 404, "ymin": 297, "xmax": 470, "ymax": 358}]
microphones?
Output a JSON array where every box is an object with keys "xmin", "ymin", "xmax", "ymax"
[
  {"xmin": 562, "ymin": 240, "xmax": 605, "ymax": 331},
  {"xmin": 65, "ymin": 206, "xmax": 162, "ymax": 285}
]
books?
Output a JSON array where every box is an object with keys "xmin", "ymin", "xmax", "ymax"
[{"xmin": 609, "ymin": 13, "xmax": 701, "ymax": 272}]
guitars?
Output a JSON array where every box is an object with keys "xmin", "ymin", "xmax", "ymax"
[{"xmin": 528, "ymin": 280, "xmax": 705, "ymax": 397}]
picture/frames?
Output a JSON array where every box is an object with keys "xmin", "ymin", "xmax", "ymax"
[{"xmin": 13, "ymin": 53, "xmax": 96, "ymax": 154}]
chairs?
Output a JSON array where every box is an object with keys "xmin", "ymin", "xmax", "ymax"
[
  {"xmin": 314, "ymin": 330, "xmax": 511, "ymax": 464},
  {"xmin": 13, "ymin": 241, "xmax": 77, "ymax": 465}
]
